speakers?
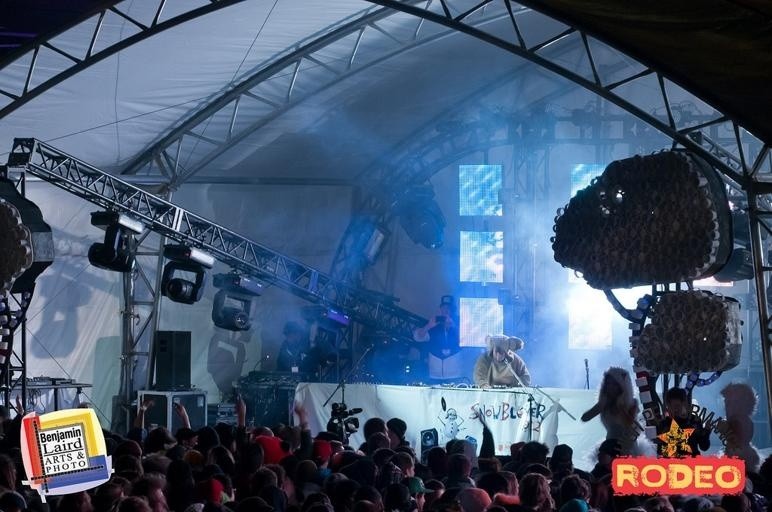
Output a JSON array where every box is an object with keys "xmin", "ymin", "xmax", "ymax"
[{"xmin": 154, "ymin": 331, "xmax": 191, "ymax": 392}]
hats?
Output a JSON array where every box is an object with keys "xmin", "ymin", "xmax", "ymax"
[{"xmin": 484, "ymin": 333, "xmax": 523, "ymax": 352}]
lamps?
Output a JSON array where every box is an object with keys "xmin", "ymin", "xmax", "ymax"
[{"xmin": 90, "ymin": 208, "xmax": 263, "ymax": 330}]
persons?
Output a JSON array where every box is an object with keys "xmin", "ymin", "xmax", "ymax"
[
  {"xmin": 0, "ymin": 366, "xmax": 771, "ymax": 511},
  {"xmin": 473, "ymin": 334, "xmax": 532, "ymax": 392},
  {"xmin": 276, "ymin": 320, "xmax": 301, "ymax": 372},
  {"xmin": 412, "ymin": 294, "xmax": 465, "ymax": 385}
]
